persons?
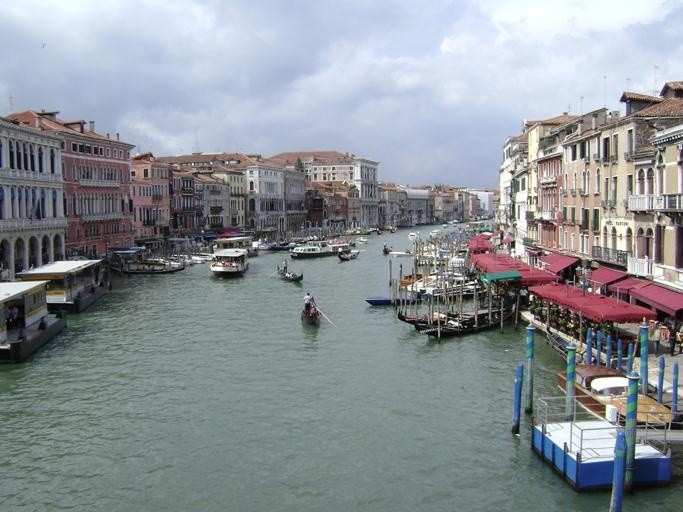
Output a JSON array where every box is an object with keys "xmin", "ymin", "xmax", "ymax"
[
  {"xmin": 302, "ymin": 292, "xmax": 312, "ymax": 316},
  {"xmin": 649, "ymin": 320, "xmax": 683, "ymax": 357},
  {"xmin": 5, "ymin": 305, "xmax": 18, "ymax": 330},
  {"xmin": 338, "ymin": 246, "xmax": 343, "ymax": 255},
  {"xmin": 282, "ymin": 259, "xmax": 287, "ymax": 273}
]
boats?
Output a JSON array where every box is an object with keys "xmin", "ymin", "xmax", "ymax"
[
  {"xmin": 300, "ymin": 310, "xmax": 322, "ymax": 327},
  {"xmin": 414, "ymin": 302, "xmax": 516, "ymax": 338},
  {"xmin": 554, "ymin": 364, "xmax": 676, "ymax": 430},
  {"xmin": 396, "ymin": 310, "xmax": 460, "ymax": 327},
  {"xmin": 276, "ymin": 265, "xmax": 303, "ymax": 282},
  {"xmin": 0, "ymin": 281, "xmax": 69, "ymax": 364},
  {"xmin": 288, "ymin": 239, "xmax": 350, "ymax": 259},
  {"xmin": 381, "ymin": 216, "xmax": 490, "ymax": 297},
  {"xmin": 336, "ymin": 251, "xmax": 359, "ymax": 261},
  {"xmin": 107, "ymin": 235, "xmax": 220, "ymax": 276},
  {"xmin": 208, "ymin": 247, "xmax": 249, "ymax": 275},
  {"xmin": 364, "ymin": 292, "xmax": 422, "ymax": 307},
  {"xmin": 14, "ymin": 259, "xmax": 113, "ymax": 317}
]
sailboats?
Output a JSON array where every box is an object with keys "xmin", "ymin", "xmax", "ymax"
[{"xmin": 251, "ymin": 215, "xmax": 384, "ymax": 249}]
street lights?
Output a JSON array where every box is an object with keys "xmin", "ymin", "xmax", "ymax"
[{"xmin": 574, "ymin": 263, "xmax": 592, "ymax": 296}]
